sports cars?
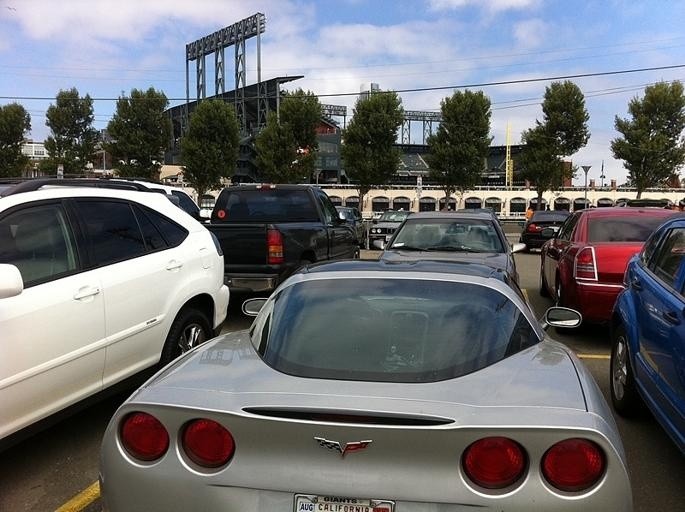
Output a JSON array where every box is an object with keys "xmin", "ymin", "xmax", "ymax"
[{"xmin": 98, "ymin": 258, "xmax": 636, "ymax": 512}]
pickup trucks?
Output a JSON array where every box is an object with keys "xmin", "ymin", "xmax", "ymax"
[{"xmin": 201, "ymin": 184, "xmax": 359, "ymax": 301}]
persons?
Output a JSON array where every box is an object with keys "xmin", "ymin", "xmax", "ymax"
[
  {"xmin": 546, "ymin": 205, "xmax": 551, "ymax": 211},
  {"xmin": 525, "ymin": 206, "xmax": 534, "ymax": 221}
]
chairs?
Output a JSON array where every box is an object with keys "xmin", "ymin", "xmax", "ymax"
[
  {"xmin": 229, "ymin": 203, "xmax": 307, "ymax": 218},
  {"xmin": 311, "ymin": 305, "xmax": 496, "ymax": 365},
  {"xmin": 416, "ymin": 226, "xmax": 491, "ymax": 246},
  {"xmin": 11, "ymin": 212, "xmax": 117, "ymax": 284}
]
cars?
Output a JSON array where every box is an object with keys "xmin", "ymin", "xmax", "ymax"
[
  {"xmin": 360, "ymin": 211, "xmax": 526, "ymax": 300},
  {"xmin": 444, "ymin": 209, "xmax": 506, "ymax": 235},
  {"xmin": 539, "ymin": 207, "xmax": 685, "ymax": 335},
  {"xmin": 365, "ymin": 210, "xmax": 424, "ymax": 251},
  {"xmin": 608, "ymin": 213, "xmax": 685, "ymax": 456},
  {"xmin": 334, "ymin": 205, "xmax": 368, "ymax": 250},
  {"xmin": 518, "ymin": 210, "xmax": 572, "ymax": 254}
]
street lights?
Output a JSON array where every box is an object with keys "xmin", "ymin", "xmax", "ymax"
[{"xmin": 581, "ymin": 166, "xmax": 592, "ymax": 209}]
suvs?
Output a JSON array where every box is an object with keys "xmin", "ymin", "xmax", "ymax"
[{"xmin": 0, "ymin": 180, "xmax": 230, "ymax": 451}]
